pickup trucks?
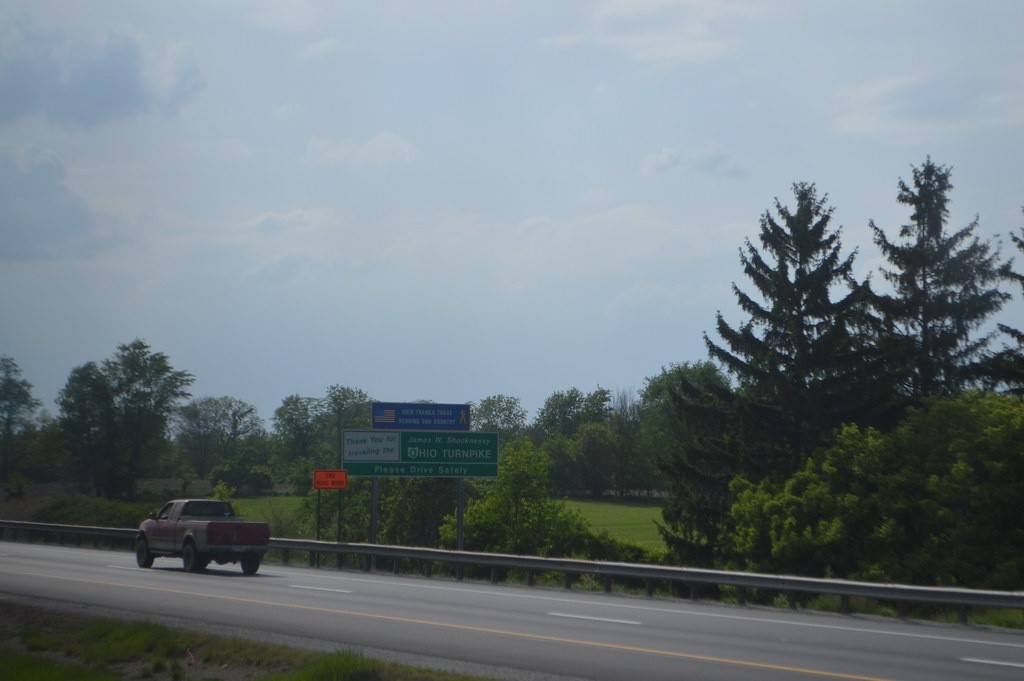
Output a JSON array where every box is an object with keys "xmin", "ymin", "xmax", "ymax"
[{"xmin": 135, "ymin": 498, "xmax": 271, "ymax": 576}]
